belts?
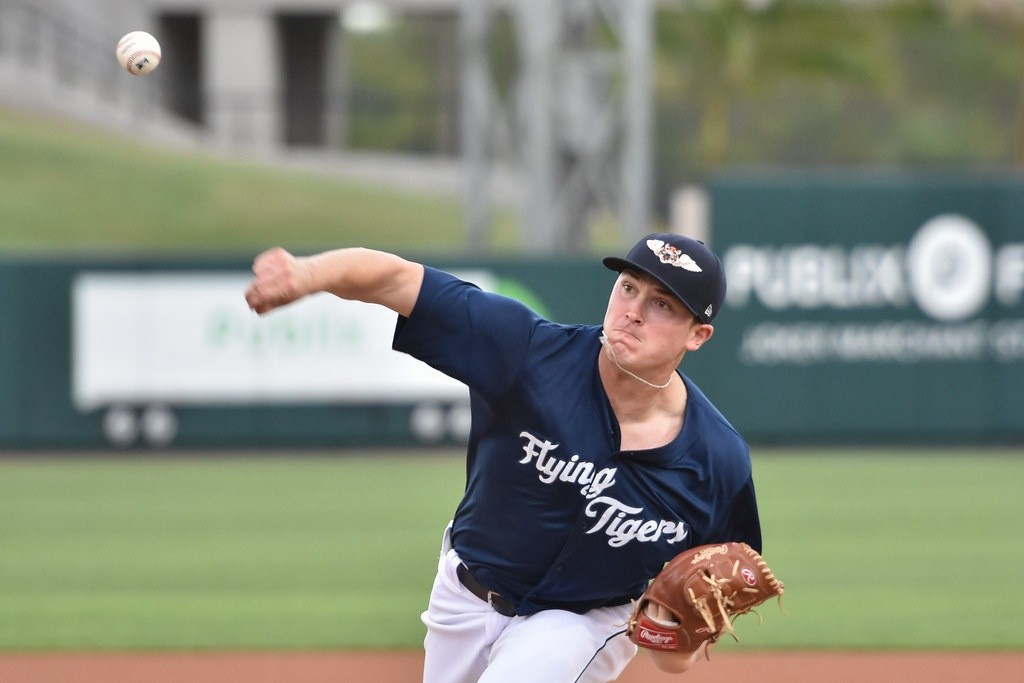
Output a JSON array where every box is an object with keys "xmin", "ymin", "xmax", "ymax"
[{"xmin": 457, "ymin": 562, "xmax": 647, "ymax": 616}]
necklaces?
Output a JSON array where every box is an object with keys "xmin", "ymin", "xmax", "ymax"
[{"xmin": 599, "ymin": 329, "xmax": 671, "ymax": 389}]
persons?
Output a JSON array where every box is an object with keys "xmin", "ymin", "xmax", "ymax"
[{"xmin": 244, "ymin": 232, "xmax": 763, "ymax": 683}]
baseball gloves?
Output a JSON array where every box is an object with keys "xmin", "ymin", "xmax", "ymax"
[{"xmin": 626, "ymin": 541, "xmax": 784, "ymax": 662}]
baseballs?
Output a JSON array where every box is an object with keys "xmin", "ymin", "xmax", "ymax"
[{"xmin": 116, "ymin": 32, "xmax": 161, "ymax": 75}]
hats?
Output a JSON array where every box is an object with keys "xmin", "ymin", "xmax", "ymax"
[{"xmin": 603, "ymin": 233, "xmax": 727, "ymax": 323}]
